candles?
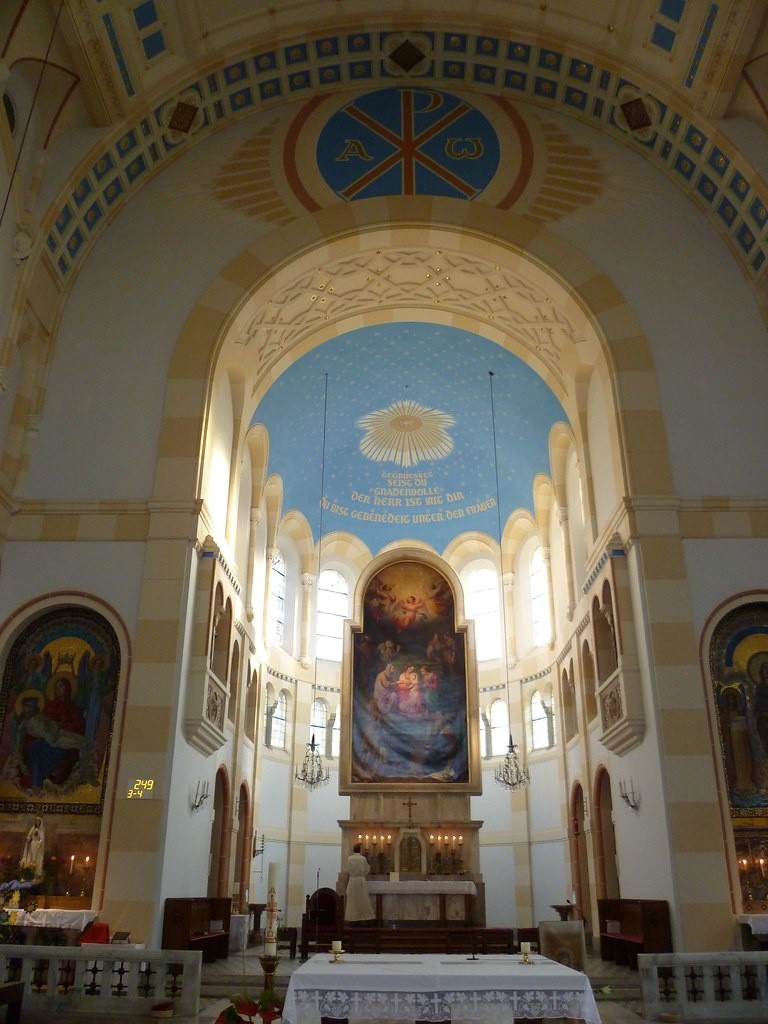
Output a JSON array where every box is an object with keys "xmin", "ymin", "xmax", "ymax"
[
  {"xmin": 521, "ymin": 942, "xmax": 530, "ymax": 952},
  {"xmin": 430, "ymin": 834, "xmax": 434, "ymax": 843},
  {"xmin": 452, "ymin": 836, "xmax": 457, "ymax": 853},
  {"xmin": 266, "ymin": 862, "xmax": 280, "ymax": 957},
  {"xmin": 332, "ymin": 941, "xmax": 341, "ymax": 950},
  {"xmin": 358, "ymin": 834, "xmax": 363, "ymax": 853},
  {"xmin": 380, "ymin": 836, "xmax": 384, "ymax": 853},
  {"xmin": 437, "ymin": 836, "xmax": 441, "ymax": 852},
  {"xmin": 459, "ymin": 836, "xmax": 463, "ymax": 844},
  {"xmin": 445, "ymin": 836, "xmax": 449, "ymax": 843},
  {"xmin": 372, "ymin": 835, "xmax": 376, "ymax": 843},
  {"xmin": 366, "ymin": 835, "xmax": 369, "ymax": 848},
  {"xmin": 387, "ymin": 835, "xmax": 391, "ymax": 843}
]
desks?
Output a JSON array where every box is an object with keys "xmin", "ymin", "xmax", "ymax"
[
  {"xmin": 81, "ymin": 943, "xmax": 146, "ymax": 991},
  {"xmin": 282, "ymin": 952, "xmax": 602, "ymax": 1024}
]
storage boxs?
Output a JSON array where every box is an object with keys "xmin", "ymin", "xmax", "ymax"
[{"xmin": 605, "ymin": 920, "xmax": 620, "ymax": 934}]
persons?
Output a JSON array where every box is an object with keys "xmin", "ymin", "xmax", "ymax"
[
  {"xmin": 345, "ymin": 844, "xmax": 375, "ymax": 926},
  {"xmin": 21, "ymin": 816, "xmax": 45, "ymax": 876}
]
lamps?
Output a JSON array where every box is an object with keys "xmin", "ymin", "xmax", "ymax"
[
  {"xmin": 85, "ymin": 856, "xmax": 89, "ymax": 867},
  {"xmin": 253, "ymin": 829, "xmax": 267, "ymax": 858},
  {"xmin": 191, "ymin": 777, "xmax": 212, "ymax": 817},
  {"xmin": 759, "ymin": 859, "xmax": 765, "ymax": 877},
  {"xmin": 742, "ymin": 859, "xmax": 747, "ymax": 871},
  {"xmin": 618, "ymin": 777, "xmax": 640, "ymax": 818},
  {"xmin": 489, "ymin": 371, "xmax": 531, "ymax": 795},
  {"xmin": 294, "ymin": 372, "xmax": 331, "ymax": 792},
  {"xmin": 69, "ymin": 855, "xmax": 74, "ymax": 874}
]
